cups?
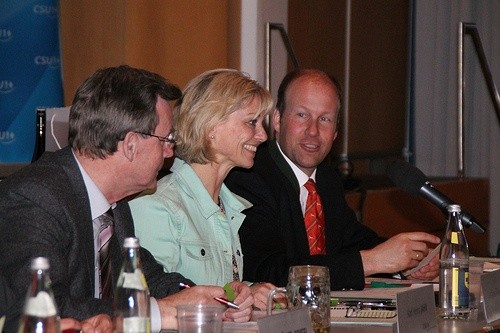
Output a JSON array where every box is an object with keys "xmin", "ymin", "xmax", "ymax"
[
  {"xmin": 177, "ymin": 304, "xmax": 223, "ymax": 333},
  {"xmin": 266, "ymin": 266, "xmax": 330, "ymax": 333}
]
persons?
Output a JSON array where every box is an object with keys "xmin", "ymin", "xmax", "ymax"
[
  {"xmin": 225, "ymin": 68, "xmax": 439, "ymax": 291},
  {"xmin": 0, "ymin": 63, "xmax": 254, "ymax": 333}
]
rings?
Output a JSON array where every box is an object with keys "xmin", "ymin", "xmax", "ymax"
[{"xmin": 416, "ymin": 252, "xmax": 419, "ymax": 260}]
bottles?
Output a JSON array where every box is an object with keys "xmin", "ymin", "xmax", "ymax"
[
  {"xmin": 440, "ymin": 204, "xmax": 470, "ymax": 321},
  {"xmin": 112, "ymin": 237, "xmax": 151, "ymax": 333},
  {"xmin": 16, "ymin": 256, "xmax": 60, "ymax": 333}
]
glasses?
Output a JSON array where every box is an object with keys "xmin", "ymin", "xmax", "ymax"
[{"xmin": 119, "ymin": 131, "xmax": 177, "ymax": 149}]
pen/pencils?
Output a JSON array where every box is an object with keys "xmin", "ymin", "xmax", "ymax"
[{"xmin": 178, "ymin": 282, "xmax": 240, "ymax": 309}]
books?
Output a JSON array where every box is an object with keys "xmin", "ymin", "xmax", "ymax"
[
  {"xmin": 220, "ymin": 309, "xmax": 282, "ymax": 332},
  {"xmin": 325, "ymin": 278, "xmax": 436, "ymax": 327}
]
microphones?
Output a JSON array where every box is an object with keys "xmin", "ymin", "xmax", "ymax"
[{"xmin": 387, "ymin": 159, "xmax": 486, "ymax": 234}]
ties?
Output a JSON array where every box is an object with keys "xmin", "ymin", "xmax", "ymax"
[
  {"xmin": 93, "ymin": 207, "xmax": 114, "ymax": 300},
  {"xmin": 303, "ymin": 179, "xmax": 327, "ymax": 256}
]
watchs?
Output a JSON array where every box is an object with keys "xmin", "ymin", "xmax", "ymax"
[{"xmin": 127, "ymin": 69, "xmax": 290, "ymax": 310}]
